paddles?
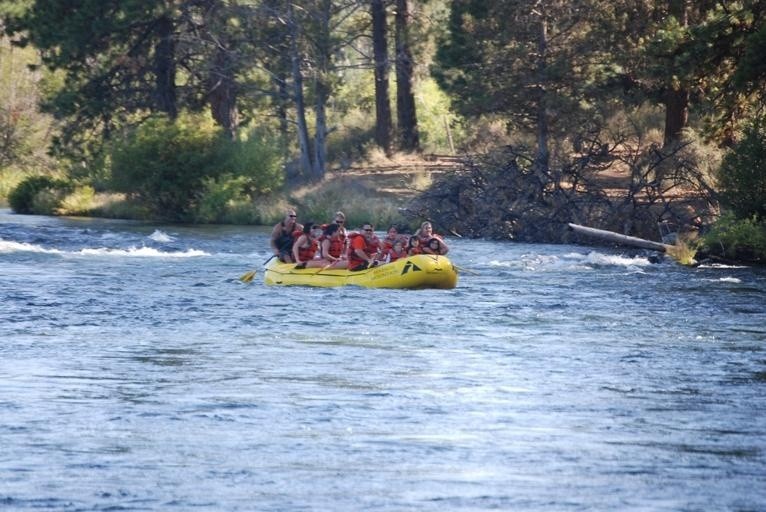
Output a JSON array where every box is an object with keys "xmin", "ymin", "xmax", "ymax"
[
  {"xmin": 239, "ymin": 255, "xmax": 277, "ymax": 283},
  {"xmin": 311, "ymin": 260, "xmax": 337, "ymax": 276}
]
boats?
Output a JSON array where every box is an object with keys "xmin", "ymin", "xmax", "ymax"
[{"xmin": 263, "ymin": 254, "xmax": 461, "ymax": 289}]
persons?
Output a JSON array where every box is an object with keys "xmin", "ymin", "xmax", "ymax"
[{"xmin": 271, "ymin": 208, "xmax": 449, "ymax": 273}]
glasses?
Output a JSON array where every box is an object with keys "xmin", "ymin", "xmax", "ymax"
[
  {"xmin": 364, "ymin": 228, "xmax": 372, "ymax": 232},
  {"xmin": 335, "ymin": 220, "xmax": 344, "ymax": 224},
  {"xmin": 287, "ymin": 214, "xmax": 297, "ymax": 218}
]
